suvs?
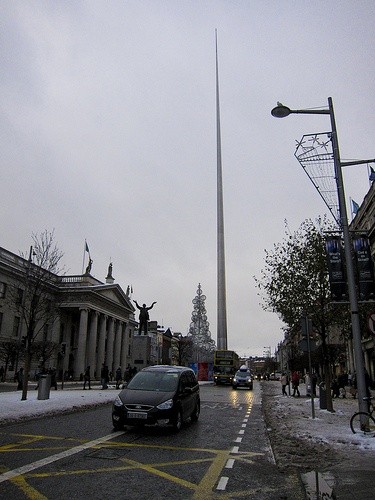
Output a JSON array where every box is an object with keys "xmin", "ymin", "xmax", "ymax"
[{"xmin": 111, "ymin": 365, "xmax": 201, "ymax": 435}]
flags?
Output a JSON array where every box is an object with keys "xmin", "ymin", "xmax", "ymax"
[
  {"xmin": 369, "ymin": 166, "xmax": 375, "ymax": 181},
  {"xmin": 86, "ymin": 243, "xmax": 90, "ymax": 259},
  {"xmin": 352, "ymin": 200, "xmax": 360, "ymax": 215}
]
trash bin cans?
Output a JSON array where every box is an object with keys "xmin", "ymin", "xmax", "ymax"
[
  {"xmin": 37, "ymin": 375, "xmax": 51, "ymax": 400},
  {"xmin": 320, "ymin": 382, "xmax": 327, "ymax": 409}
]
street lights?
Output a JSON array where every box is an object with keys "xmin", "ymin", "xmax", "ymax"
[
  {"xmin": 13, "ymin": 245, "xmax": 37, "ymax": 383},
  {"xmin": 264, "ymin": 346, "xmax": 271, "ymax": 374},
  {"xmin": 269, "ymin": 96, "xmax": 375, "ymax": 432}
]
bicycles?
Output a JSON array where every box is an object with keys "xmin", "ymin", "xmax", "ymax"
[{"xmin": 349, "ymin": 396, "xmax": 375, "ymax": 438}]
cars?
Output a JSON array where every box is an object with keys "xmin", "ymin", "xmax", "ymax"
[
  {"xmin": 265, "ymin": 372, "xmax": 280, "ymax": 381},
  {"xmin": 232, "ymin": 371, "xmax": 255, "ymax": 390}
]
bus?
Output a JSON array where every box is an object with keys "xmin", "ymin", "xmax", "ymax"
[{"xmin": 212, "ymin": 349, "xmax": 241, "ymax": 385}]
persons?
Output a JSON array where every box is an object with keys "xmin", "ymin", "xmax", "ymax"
[
  {"xmin": 303, "ymin": 367, "xmax": 372, "ymax": 397},
  {"xmin": 83, "ymin": 365, "xmax": 92, "ymax": 390},
  {"xmin": 291, "ymin": 372, "xmax": 301, "ymax": 397},
  {"xmin": 17, "ymin": 367, "xmax": 24, "ymax": 390},
  {"xmin": 34, "ymin": 366, "xmax": 58, "ymax": 390},
  {"xmin": 133, "ymin": 300, "xmax": 157, "ymax": 335},
  {"xmin": 0, "ymin": 366, "xmax": 5, "ymax": 382},
  {"xmin": 101, "ymin": 363, "xmax": 138, "ymax": 391},
  {"xmin": 281, "ymin": 373, "xmax": 287, "ymax": 396}
]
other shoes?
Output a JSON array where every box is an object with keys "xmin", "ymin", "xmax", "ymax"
[
  {"xmin": 291, "ymin": 394, "xmax": 295, "ymax": 397},
  {"xmin": 297, "ymin": 393, "xmax": 300, "ymax": 397},
  {"xmin": 89, "ymin": 388, "xmax": 92, "ymax": 390},
  {"xmin": 83, "ymin": 388, "xmax": 87, "ymax": 390}
]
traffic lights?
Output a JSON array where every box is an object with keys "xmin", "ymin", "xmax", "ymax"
[
  {"xmin": 21, "ymin": 336, "xmax": 28, "ymax": 348},
  {"xmin": 61, "ymin": 344, "xmax": 67, "ymax": 355}
]
